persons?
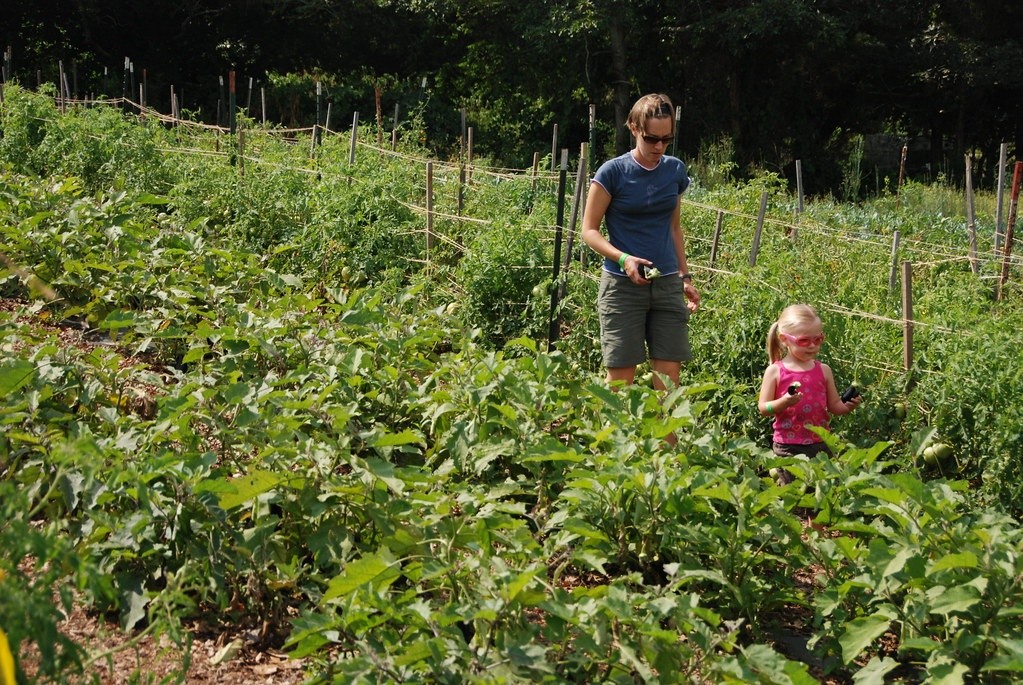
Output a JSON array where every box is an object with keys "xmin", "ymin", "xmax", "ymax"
[
  {"xmin": 580, "ymin": 94, "xmax": 700, "ymax": 447},
  {"xmin": 758, "ymin": 304, "xmax": 861, "ymax": 534}
]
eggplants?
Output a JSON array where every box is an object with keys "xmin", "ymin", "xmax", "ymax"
[
  {"xmin": 840, "ymin": 380, "xmax": 861, "ymax": 403},
  {"xmin": 638, "ymin": 263, "xmax": 661, "ymax": 279},
  {"xmin": 787, "ymin": 381, "xmax": 801, "ymax": 395}
]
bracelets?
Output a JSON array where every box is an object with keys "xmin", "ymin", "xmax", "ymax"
[
  {"xmin": 617, "ymin": 252, "xmax": 628, "ymax": 272},
  {"xmin": 767, "ymin": 402, "xmax": 775, "ymax": 416},
  {"xmin": 682, "ymin": 274, "xmax": 691, "ymax": 282}
]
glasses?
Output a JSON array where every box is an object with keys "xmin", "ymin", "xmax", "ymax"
[
  {"xmin": 782, "ymin": 332, "xmax": 824, "ymax": 347},
  {"xmin": 641, "ymin": 133, "xmax": 675, "ymax": 144}
]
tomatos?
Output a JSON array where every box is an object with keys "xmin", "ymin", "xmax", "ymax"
[
  {"xmin": 892, "ymin": 403, "xmax": 906, "ymax": 418},
  {"xmin": 923, "ymin": 443, "xmax": 952, "ymax": 464},
  {"xmin": 214, "ymin": 209, "xmax": 655, "ymax": 388}
]
